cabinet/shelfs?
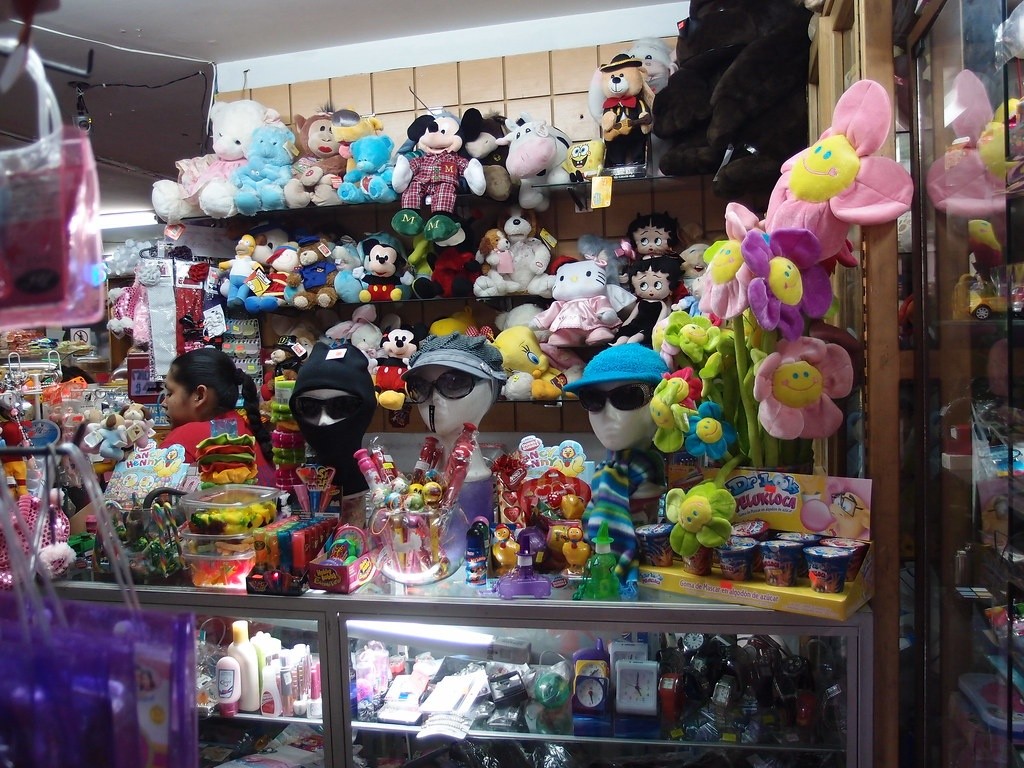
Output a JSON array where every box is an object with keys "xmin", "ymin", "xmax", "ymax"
[
  {"xmin": 178, "ymin": 169, "xmax": 722, "ymax": 434},
  {"xmin": 42, "ymin": 579, "xmax": 872, "ymax": 768}
]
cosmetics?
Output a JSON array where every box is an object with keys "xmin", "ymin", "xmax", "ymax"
[{"xmin": 212, "ymin": 618, "xmax": 322, "ymax": 720}]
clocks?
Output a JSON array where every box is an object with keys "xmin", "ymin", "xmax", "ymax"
[{"xmin": 573, "ymin": 640, "xmax": 659, "ymax": 715}]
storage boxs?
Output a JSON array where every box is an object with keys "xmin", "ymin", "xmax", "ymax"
[
  {"xmin": 636, "ymin": 465, "xmax": 874, "ymax": 622},
  {"xmin": 69, "ymin": 484, "xmax": 383, "ymax": 594},
  {"xmin": 944, "ymin": 691, "xmax": 1024, "ymax": 768}
]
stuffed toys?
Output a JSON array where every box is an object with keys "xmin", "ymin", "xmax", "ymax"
[
  {"xmin": 649, "ymin": 0, "xmax": 823, "ymax": 198},
  {"xmin": 391, "ymin": 84, "xmax": 521, "ymax": 242},
  {"xmin": 495, "ymin": 112, "xmax": 593, "ymax": 213},
  {"xmin": 216, "ymin": 220, "xmax": 637, "ymax": 430},
  {"xmin": 925, "ymin": 70, "xmax": 1006, "ymax": 267},
  {"xmin": 331, "ymin": 105, "xmax": 385, "ymax": 190},
  {"xmin": 285, "ymin": 104, "xmax": 347, "ymax": 208},
  {"xmin": 336, "ymin": 135, "xmax": 398, "ymax": 204},
  {"xmin": 81, "ymin": 402, "xmax": 154, "ymax": 461},
  {"xmin": 588, "ymin": 36, "xmax": 680, "ymax": 176},
  {"xmin": 231, "ymin": 125, "xmax": 296, "ymax": 217},
  {"xmin": 567, "ymin": 139, "xmax": 605, "ymax": 183},
  {"xmin": 649, "ymin": 80, "xmax": 914, "ymax": 557},
  {"xmin": 151, "ymin": 100, "xmax": 287, "ymax": 226}
]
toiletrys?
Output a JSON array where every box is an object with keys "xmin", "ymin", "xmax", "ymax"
[{"xmin": 216, "ymin": 618, "xmax": 393, "ymax": 719}]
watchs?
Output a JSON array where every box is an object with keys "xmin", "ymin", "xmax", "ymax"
[{"xmin": 657, "ymin": 632, "xmax": 847, "ymax": 745}]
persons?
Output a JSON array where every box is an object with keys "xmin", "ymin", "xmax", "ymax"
[
  {"xmin": 400, "ymin": 332, "xmax": 505, "ymax": 484},
  {"xmin": 604, "ymin": 209, "xmax": 712, "ymax": 348},
  {"xmin": 289, "ymin": 342, "xmax": 376, "ymax": 502},
  {"xmin": 61, "ymin": 347, "xmax": 278, "ymax": 531},
  {"xmin": 561, "ymin": 342, "xmax": 671, "ymax": 499},
  {"xmin": 0, "ymin": 407, "xmax": 35, "ymax": 504}
]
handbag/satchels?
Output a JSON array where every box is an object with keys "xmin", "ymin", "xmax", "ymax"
[
  {"xmin": 1, "ymin": 443, "xmax": 198, "ymax": 764},
  {"xmin": 0, "ymin": 36, "xmax": 104, "ymax": 325}
]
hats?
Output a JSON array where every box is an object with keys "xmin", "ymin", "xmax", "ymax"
[
  {"xmin": 401, "ymin": 331, "xmax": 507, "ymax": 405},
  {"xmin": 289, "ymin": 342, "xmax": 377, "ymax": 497},
  {"xmin": 563, "ymin": 343, "xmax": 670, "ymax": 394}
]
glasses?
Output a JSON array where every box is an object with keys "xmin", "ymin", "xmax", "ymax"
[
  {"xmin": 576, "ymin": 382, "xmax": 655, "ymax": 412},
  {"xmin": 407, "ymin": 369, "xmax": 485, "ymax": 404},
  {"xmin": 294, "ymin": 395, "xmax": 358, "ymax": 419}
]
